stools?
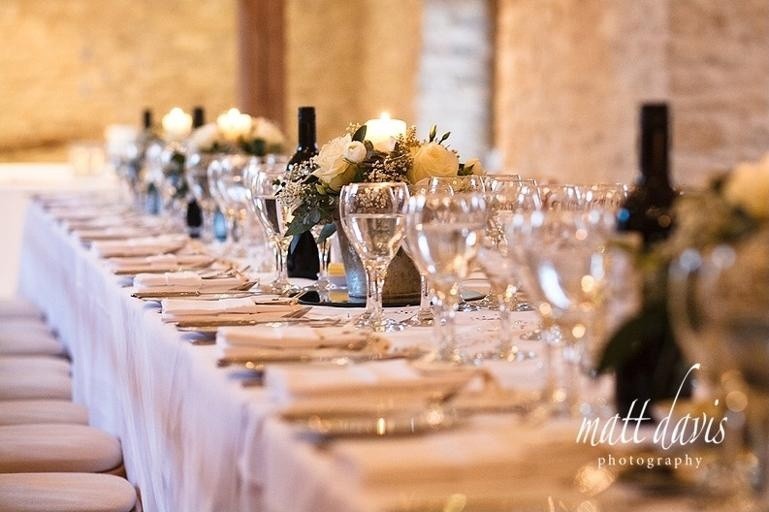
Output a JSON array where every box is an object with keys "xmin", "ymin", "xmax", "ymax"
[{"xmin": 2, "ymin": 298, "xmax": 149, "ymax": 511}]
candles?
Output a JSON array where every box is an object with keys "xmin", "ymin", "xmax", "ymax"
[
  {"xmin": 363, "ymin": 112, "xmax": 409, "ymax": 155},
  {"xmin": 218, "ymin": 106, "xmax": 254, "ymax": 141},
  {"xmin": 162, "ymin": 106, "xmax": 195, "ymax": 140}
]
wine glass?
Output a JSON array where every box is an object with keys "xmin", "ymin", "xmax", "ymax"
[
  {"xmin": 340, "ymin": 176, "xmax": 767, "ymax": 512},
  {"xmin": 69, "ymin": 132, "xmax": 338, "ymax": 328}
]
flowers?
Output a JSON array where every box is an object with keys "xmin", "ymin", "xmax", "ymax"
[
  {"xmin": 184, "ymin": 113, "xmax": 289, "ymax": 160},
  {"xmin": 277, "ymin": 134, "xmax": 491, "ymax": 244},
  {"xmin": 597, "ymin": 153, "xmax": 769, "ymax": 424}
]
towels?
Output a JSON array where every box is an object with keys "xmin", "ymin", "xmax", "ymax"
[{"xmin": 40, "ymin": 190, "xmax": 476, "ymax": 432}]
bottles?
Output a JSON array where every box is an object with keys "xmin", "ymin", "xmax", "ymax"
[
  {"xmin": 133, "ymin": 107, "xmax": 162, "ymax": 213},
  {"xmin": 285, "ymin": 105, "xmax": 337, "ymax": 298},
  {"xmin": 608, "ymin": 99, "xmax": 701, "ymax": 424},
  {"xmin": 183, "ymin": 101, "xmax": 208, "ymax": 234}
]
